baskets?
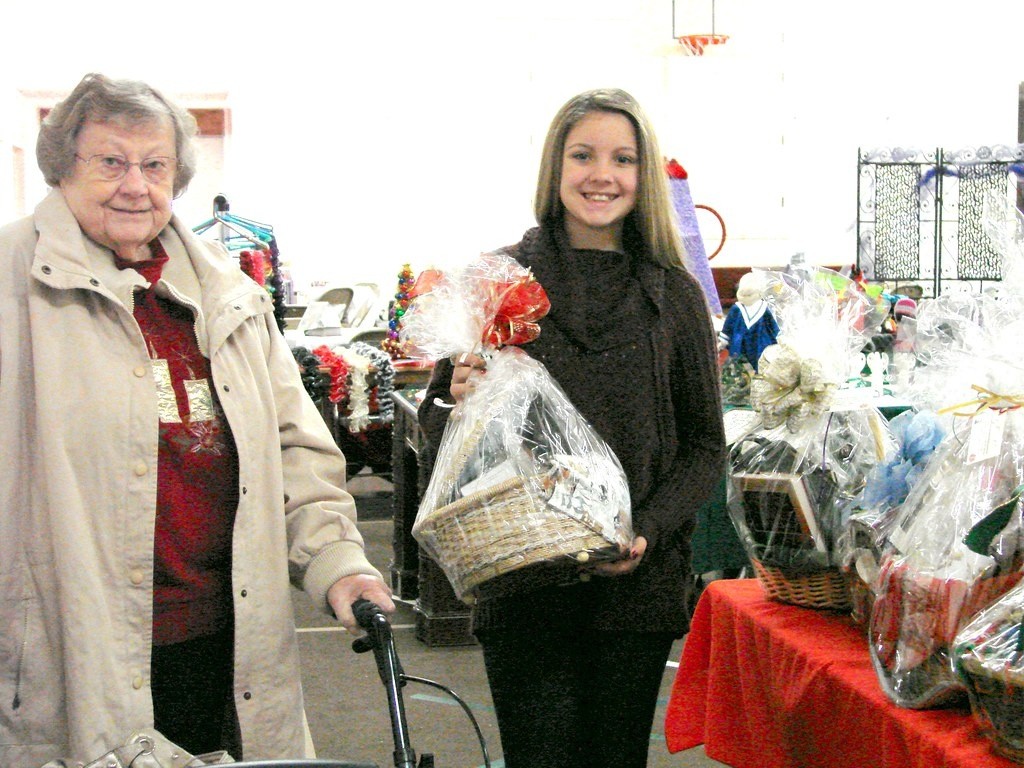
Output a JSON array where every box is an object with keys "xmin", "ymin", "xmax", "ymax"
[
  {"xmin": 958, "ymin": 647, "xmax": 1024, "ymax": 763},
  {"xmin": 871, "ymin": 422, "xmax": 1023, "ymax": 655},
  {"xmin": 411, "ymin": 386, "xmax": 613, "ymax": 603},
  {"xmin": 726, "ymin": 468, "xmax": 861, "ymax": 610}
]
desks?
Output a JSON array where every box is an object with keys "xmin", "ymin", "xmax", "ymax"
[
  {"xmin": 664, "ymin": 576, "xmax": 1024, "ymax": 768},
  {"xmin": 297, "ymin": 362, "xmax": 431, "ymax": 602}
]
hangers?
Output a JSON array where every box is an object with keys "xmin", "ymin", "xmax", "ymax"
[{"xmin": 189, "ymin": 194, "xmax": 274, "ymax": 253}]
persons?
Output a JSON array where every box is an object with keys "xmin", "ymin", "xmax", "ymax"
[
  {"xmin": 718, "ymin": 271, "xmax": 781, "ymax": 373},
  {"xmin": 0, "ymin": 74, "xmax": 397, "ymax": 768},
  {"xmin": 416, "ymin": 87, "xmax": 727, "ymax": 768}
]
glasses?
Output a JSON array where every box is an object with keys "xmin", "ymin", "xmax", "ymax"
[{"xmin": 71, "ymin": 151, "xmax": 180, "ymax": 184}]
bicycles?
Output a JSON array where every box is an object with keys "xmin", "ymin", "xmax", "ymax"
[{"xmin": 195, "ymin": 592, "xmax": 491, "ymax": 768}]
